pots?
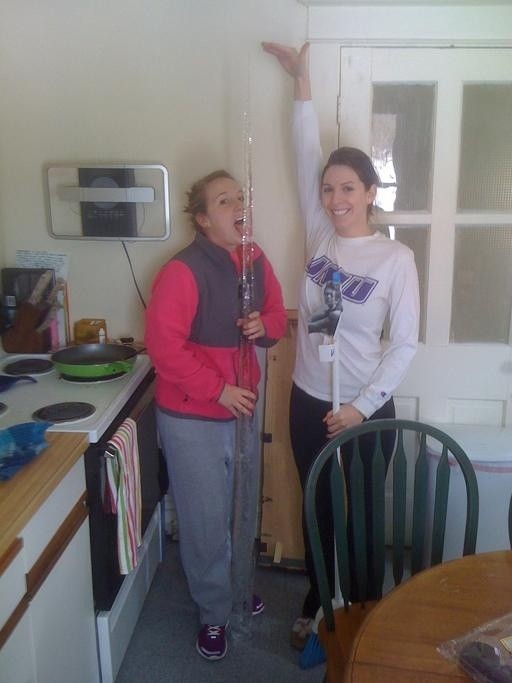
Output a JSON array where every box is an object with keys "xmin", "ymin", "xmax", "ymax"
[{"xmin": 53, "ymin": 342, "xmax": 148, "ymax": 378}]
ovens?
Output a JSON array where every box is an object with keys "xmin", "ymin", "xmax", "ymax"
[{"xmin": 90, "ymin": 367, "xmax": 163, "ymax": 609}]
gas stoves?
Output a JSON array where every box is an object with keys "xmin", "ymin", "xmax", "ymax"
[{"xmin": 0, "ymin": 352, "xmax": 156, "ymax": 439}]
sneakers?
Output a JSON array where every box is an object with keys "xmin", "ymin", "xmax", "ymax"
[
  {"xmin": 196, "ymin": 620, "xmax": 229, "ymax": 659},
  {"xmin": 292, "ymin": 619, "xmax": 314, "ymax": 649},
  {"xmin": 252, "ymin": 594, "xmax": 265, "ymax": 616}
]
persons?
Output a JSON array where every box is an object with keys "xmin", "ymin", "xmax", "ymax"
[
  {"xmin": 260, "ymin": 39, "xmax": 421, "ymax": 655},
  {"xmin": 145, "ymin": 165, "xmax": 289, "ymax": 664}
]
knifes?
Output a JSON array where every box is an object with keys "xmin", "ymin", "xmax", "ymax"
[{"xmin": 32, "ymin": 269, "xmax": 65, "ymax": 333}]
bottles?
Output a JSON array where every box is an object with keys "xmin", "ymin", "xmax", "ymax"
[{"xmin": 98, "ymin": 328, "xmax": 106, "ymax": 344}]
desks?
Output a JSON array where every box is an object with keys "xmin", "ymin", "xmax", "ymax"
[{"xmin": 345, "ymin": 549, "xmax": 512, "ymax": 683}]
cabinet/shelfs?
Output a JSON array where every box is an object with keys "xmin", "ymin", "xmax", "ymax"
[{"xmin": 0, "ymin": 431, "xmax": 102, "ymax": 683}]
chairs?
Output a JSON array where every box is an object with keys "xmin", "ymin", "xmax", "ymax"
[{"xmin": 304, "ymin": 417, "xmax": 480, "ymax": 683}]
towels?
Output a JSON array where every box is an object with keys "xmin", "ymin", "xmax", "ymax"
[{"xmin": 108, "ymin": 416, "xmax": 144, "ymax": 574}]
